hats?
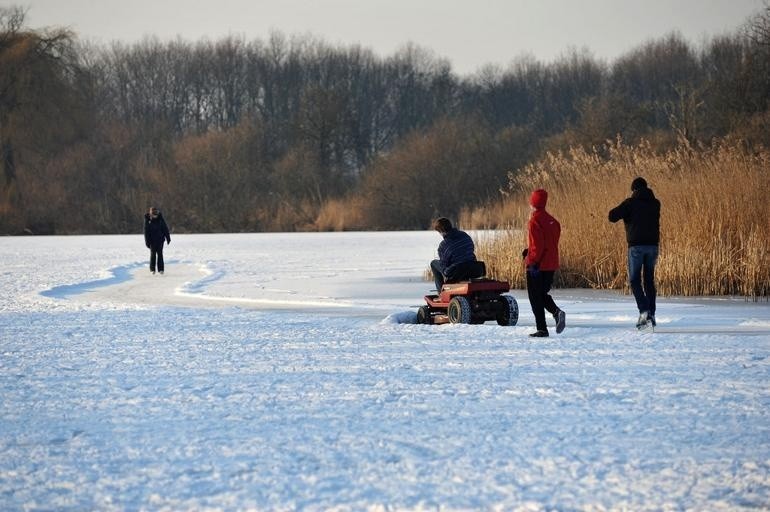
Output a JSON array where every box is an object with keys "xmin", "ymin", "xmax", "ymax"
[{"xmin": 530, "ymin": 188, "xmax": 549, "ymax": 208}]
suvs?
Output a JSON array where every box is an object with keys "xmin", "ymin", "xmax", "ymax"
[{"xmin": 417, "ymin": 261, "xmax": 519, "ymax": 326}]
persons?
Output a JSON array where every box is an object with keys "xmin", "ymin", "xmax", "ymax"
[
  {"xmin": 430, "ymin": 217, "xmax": 479, "ymax": 294},
  {"xmin": 608, "ymin": 177, "xmax": 662, "ymax": 329},
  {"xmin": 143, "ymin": 206, "xmax": 170, "ymax": 276},
  {"xmin": 521, "ymin": 188, "xmax": 566, "ymax": 337}
]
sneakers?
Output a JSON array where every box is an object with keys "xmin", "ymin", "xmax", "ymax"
[
  {"xmin": 433, "ymin": 293, "xmax": 441, "ymax": 303},
  {"xmin": 151, "ymin": 270, "xmax": 164, "ymax": 275},
  {"xmin": 529, "ymin": 330, "xmax": 549, "ymax": 337},
  {"xmin": 556, "ymin": 310, "xmax": 565, "ymax": 334},
  {"xmin": 636, "ymin": 311, "xmax": 656, "ymax": 331}
]
